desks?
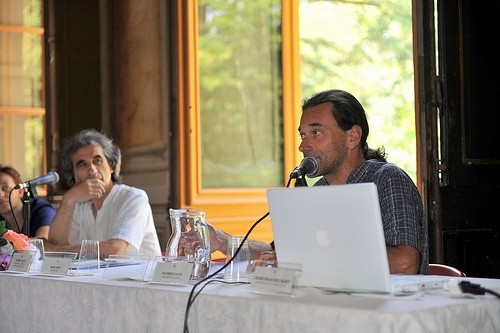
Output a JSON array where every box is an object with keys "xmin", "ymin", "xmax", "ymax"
[{"xmin": 0, "ymin": 259, "xmax": 500, "ymax": 333}]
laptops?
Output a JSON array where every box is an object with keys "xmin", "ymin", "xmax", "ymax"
[{"xmin": 267, "ymin": 182, "xmax": 450, "ymax": 295}]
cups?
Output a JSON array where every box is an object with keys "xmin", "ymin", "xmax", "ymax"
[
  {"xmin": 223, "ymin": 236, "xmax": 252, "ymax": 285},
  {"xmin": 27, "ymin": 239, "xmax": 45, "ymax": 272},
  {"xmin": 76, "ymin": 240, "xmax": 101, "ymax": 276}
]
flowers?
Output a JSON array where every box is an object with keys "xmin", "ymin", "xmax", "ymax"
[{"xmin": 0, "ymin": 220, "xmax": 30, "ymax": 263}]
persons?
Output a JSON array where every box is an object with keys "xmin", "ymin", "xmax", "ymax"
[
  {"xmin": 179, "ymin": 89, "xmax": 430, "ymax": 274},
  {"xmin": 37, "ymin": 130, "xmax": 164, "ymax": 260},
  {"xmin": 0, "ymin": 166, "xmax": 56, "ymax": 241}
]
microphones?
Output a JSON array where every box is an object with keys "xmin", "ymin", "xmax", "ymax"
[{"xmin": 13, "ymin": 171, "xmax": 60, "ymax": 190}]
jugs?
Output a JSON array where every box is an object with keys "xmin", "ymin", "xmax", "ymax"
[{"xmin": 166, "ymin": 208, "xmax": 211, "ymax": 283}]
